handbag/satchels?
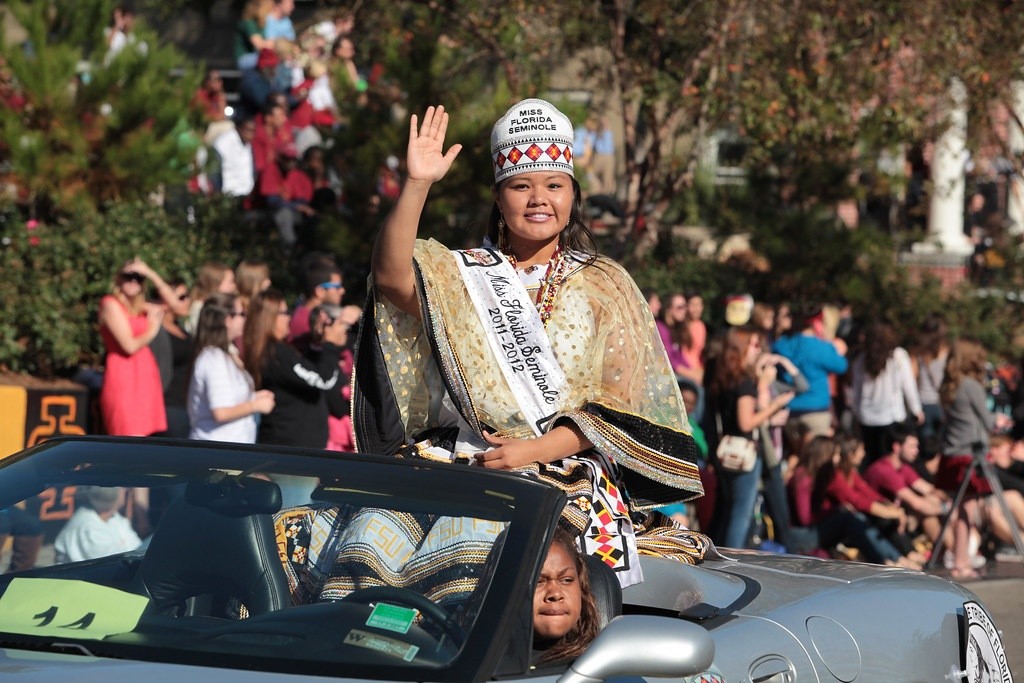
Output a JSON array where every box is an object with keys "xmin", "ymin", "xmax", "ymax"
[{"xmin": 716, "ymin": 434, "xmax": 757, "ymax": 472}]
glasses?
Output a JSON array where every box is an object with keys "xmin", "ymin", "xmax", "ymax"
[
  {"xmin": 229, "ymin": 311, "xmax": 244, "ymax": 317},
  {"xmin": 121, "ymin": 273, "xmax": 144, "ymax": 283},
  {"xmin": 277, "ymin": 309, "xmax": 292, "ymax": 315},
  {"xmin": 320, "ymin": 282, "xmax": 342, "ymax": 290}
]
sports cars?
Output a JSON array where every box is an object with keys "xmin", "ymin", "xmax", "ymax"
[{"xmin": 0, "ymin": 431, "xmax": 1010, "ymax": 683}]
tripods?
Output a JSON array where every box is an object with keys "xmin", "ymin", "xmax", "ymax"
[{"xmin": 921, "ymin": 440, "xmax": 1024, "ymax": 574}]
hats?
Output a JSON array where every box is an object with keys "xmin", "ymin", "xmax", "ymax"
[{"xmin": 491, "ymin": 99, "xmax": 574, "ymax": 183}]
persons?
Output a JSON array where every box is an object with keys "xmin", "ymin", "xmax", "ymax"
[
  {"xmin": 289, "ymin": 259, "xmax": 366, "ymax": 451},
  {"xmin": 0, "ymin": 0, "xmax": 406, "ymax": 251},
  {"xmin": 152, "ymin": 280, "xmax": 193, "ymax": 438},
  {"xmin": 188, "ymin": 297, "xmax": 277, "ymax": 444},
  {"xmin": 368, "ymin": 99, "xmax": 705, "ymax": 588},
  {"xmin": 242, "ymin": 289, "xmax": 346, "ymax": 449},
  {"xmin": 54, "ymin": 485, "xmax": 142, "ymax": 563},
  {"xmin": 455, "ymin": 523, "xmax": 600, "ymax": 666},
  {"xmin": 641, "ymin": 290, "xmax": 1024, "ymax": 577},
  {"xmin": 185, "ymin": 261, "xmax": 272, "ymax": 360},
  {"xmin": 0, "ymin": 506, "xmax": 44, "ymax": 572},
  {"xmin": 965, "ymin": 195, "xmax": 987, "ymax": 284},
  {"xmin": 97, "ymin": 256, "xmax": 175, "ymax": 438}
]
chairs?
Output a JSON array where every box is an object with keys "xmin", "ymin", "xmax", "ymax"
[
  {"xmin": 128, "ymin": 488, "xmax": 289, "ymax": 631},
  {"xmin": 581, "ymin": 553, "xmax": 622, "ymax": 632}
]
